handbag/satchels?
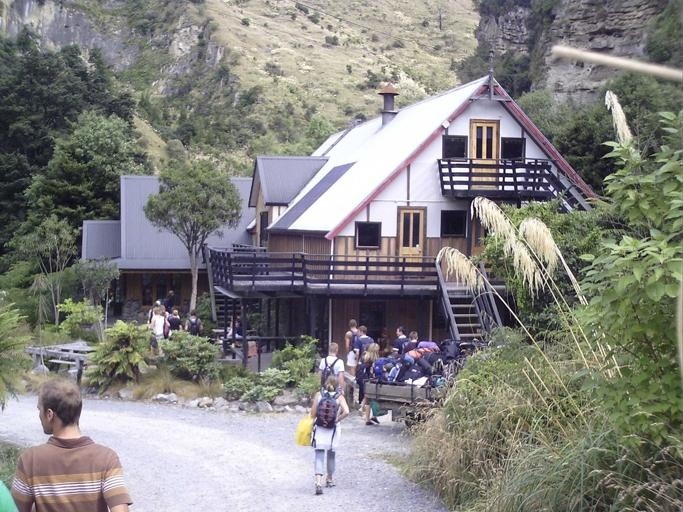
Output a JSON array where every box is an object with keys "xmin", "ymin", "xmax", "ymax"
[
  {"xmin": 163, "ymin": 323, "xmax": 169, "ymax": 338},
  {"xmin": 373, "ymin": 341, "xmax": 462, "ymax": 387},
  {"xmin": 297, "ymin": 414, "xmax": 313, "ymax": 446}
]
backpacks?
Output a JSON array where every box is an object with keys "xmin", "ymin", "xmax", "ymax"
[
  {"xmin": 350, "ymin": 329, "xmax": 361, "ymax": 349},
  {"xmin": 356, "ymin": 361, "xmax": 370, "ymax": 386},
  {"xmin": 317, "ymin": 388, "xmax": 342, "ymax": 428},
  {"xmin": 321, "ymin": 358, "xmax": 338, "ymax": 387},
  {"xmin": 188, "ymin": 318, "xmax": 199, "ymax": 336}
]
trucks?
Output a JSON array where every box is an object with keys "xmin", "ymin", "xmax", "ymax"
[{"xmin": 363, "ymin": 337, "xmax": 492, "ymax": 428}]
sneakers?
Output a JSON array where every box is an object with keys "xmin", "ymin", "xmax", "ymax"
[
  {"xmin": 327, "ymin": 480, "xmax": 335, "ymax": 488},
  {"xmin": 359, "ymin": 404, "xmax": 379, "ymax": 425},
  {"xmin": 316, "ymin": 484, "xmax": 323, "ymax": 494}
]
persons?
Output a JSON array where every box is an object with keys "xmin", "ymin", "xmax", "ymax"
[
  {"xmin": 310, "ymin": 375, "xmax": 350, "ymax": 495},
  {"xmin": 343, "ymin": 318, "xmax": 420, "ymax": 425},
  {"xmin": 146, "ymin": 290, "xmax": 243, "ymax": 357},
  {"xmin": 10, "ymin": 378, "xmax": 134, "ymax": 512},
  {"xmin": 317, "ymin": 342, "xmax": 345, "ymax": 385}
]
